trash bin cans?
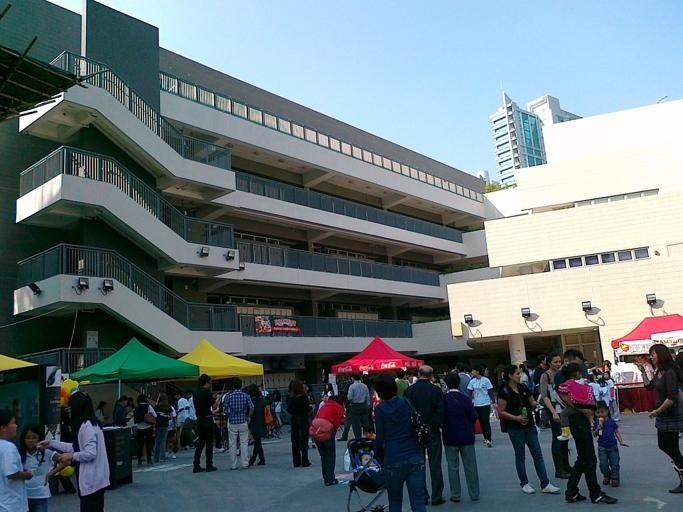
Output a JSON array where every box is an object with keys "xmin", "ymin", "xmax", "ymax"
[{"xmin": 103, "ymin": 427, "xmax": 132, "ymax": 489}]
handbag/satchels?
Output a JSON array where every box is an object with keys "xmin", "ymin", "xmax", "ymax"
[
  {"xmin": 143, "ymin": 413, "xmax": 157, "ymax": 424},
  {"xmin": 499, "ymin": 414, "xmax": 509, "ymax": 434},
  {"xmin": 265, "ymin": 406, "xmax": 276, "ymax": 428},
  {"xmin": 412, "ymin": 412, "xmax": 435, "ymax": 448},
  {"xmin": 306, "ymin": 417, "xmax": 337, "ymax": 443}
]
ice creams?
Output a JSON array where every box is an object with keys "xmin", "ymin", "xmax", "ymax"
[{"xmin": 598, "ymin": 417, "xmax": 604, "ymax": 425}]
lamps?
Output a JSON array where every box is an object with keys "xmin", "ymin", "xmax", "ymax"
[
  {"xmin": 582, "ymin": 300, "xmax": 591, "ymax": 312},
  {"xmin": 26, "ymin": 282, "xmax": 42, "ymax": 296},
  {"xmin": 98, "ymin": 278, "xmax": 114, "ymax": 297},
  {"xmin": 70, "ymin": 277, "xmax": 90, "ymax": 296},
  {"xmin": 237, "ymin": 263, "xmax": 245, "ymax": 271},
  {"xmin": 197, "ymin": 246, "xmax": 210, "ymax": 258},
  {"xmin": 223, "ymin": 249, "xmax": 235, "ymax": 262},
  {"xmin": 646, "ymin": 293, "xmax": 656, "ymax": 305},
  {"xmin": 463, "ymin": 314, "xmax": 473, "ymax": 324},
  {"xmin": 520, "ymin": 307, "xmax": 530, "ymax": 318}
]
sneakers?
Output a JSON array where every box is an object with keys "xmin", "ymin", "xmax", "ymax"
[
  {"xmin": 553, "ymin": 470, "xmax": 573, "ymax": 480},
  {"xmin": 138, "ymin": 445, "xmax": 266, "ymax": 474},
  {"xmin": 540, "ymin": 482, "xmax": 561, "ymax": 494},
  {"xmin": 520, "ymin": 483, "xmax": 537, "ymax": 495},
  {"xmin": 449, "ymin": 494, "xmax": 460, "ymax": 503},
  {"xmin": 290, "ymin": 436, "xmax": 349, "ymax": 487},
  {"xmin": 431, "ymin": 499, "xmax": 446, "ymax": 505},
  {"xmin": 601, "ymin": 475, "xmax": 621, "ymax": 487},
  {"xmin": 588, "ymin": 490, "xmax": 619, "ymax": 505},
  {"xmin": 565, "ymin": 491, "xmax": 588, "ymax": 503},
  {"xmin": 486, "ymin": 440, "xmax": 492, "ymax": 448}
]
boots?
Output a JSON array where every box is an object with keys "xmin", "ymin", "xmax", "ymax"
[{"xmin": 667, "ymin": 465, "xmax": 683, "ymax": 494}]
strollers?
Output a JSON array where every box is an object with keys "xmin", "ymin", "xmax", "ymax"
[{"xmin": 346, "ymin": 437, "xmax": 391, "ymax": 512}]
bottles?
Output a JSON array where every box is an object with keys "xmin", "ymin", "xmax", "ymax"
[
  {"xmin": 648, "ymin": 413, "xmax": 654, "ymax": 429},
  {"xmin": 521, "ymin": 407, "xmax": 528, "ymax": 425},
  {"xmin": 31, "ymin": 467, "xmax": 45, "ymax": 477}
]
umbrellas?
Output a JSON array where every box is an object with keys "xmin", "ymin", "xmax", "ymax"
[
  {"xmin": 68, "ymin": 337, "xmax": 198, "ymax": 403},
  {"xmin": 0, "ymin": 353, "xmax": 40, "ymax": 375},
  {"xmin": 331, "ymin": 336, "xmax": 422, "ymax": 374},
  {"xmin": 176, "ymin": 338, "xmax": 267, "ymax": 386}
]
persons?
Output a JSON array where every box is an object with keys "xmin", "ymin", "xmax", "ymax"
[
  {"xmin": 558, "ymin": 362, "xmax": 597, "ymax": 442},
  {"xmin": 369, "ymin": 373, "xmax": 431, "ymax": 512},
  {"xmin": 491, "ymin": 364, "xmax": 558, "ymax": 493},
  {"xmin": 18, "ymin": 423, "xmax": 68, "ymax": 511},
  {"xmin": 402, "ymin": 364, "xmax": 448, "ymax": 506},
  {"xmin": 637, "ymin": 342, "xmax": 682, "ymax": 495},
  {"xmin": 34, "ymin": 389, "xmax": 110, "ymax": 510},
  {"xmin": 0, "ymin": 407, "xmax": 32, "ymax": 512},
  {"xmin": 91, "ymin": 372, "xmax": 283, "ymax": 471},
  {"xmin": 438, "ymin": 370, "xmax": 482, "ymax": 502},
  {"xmin": 554, "ymin": 350, "xmax": 619, "ymax": 506}
]
